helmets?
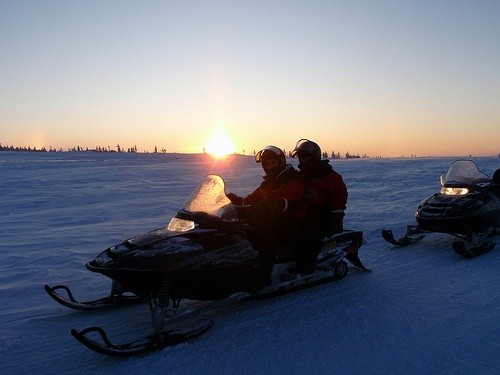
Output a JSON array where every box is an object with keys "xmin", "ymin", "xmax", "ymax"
[
  {"xmin": 288, "ymin": 139, "xmax": 321, "ymax": 160},
  {"xmin": 255, "ymin": 146, "xmax": 286, "ymax": 170}
]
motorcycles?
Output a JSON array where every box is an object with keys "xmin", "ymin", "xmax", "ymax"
[
  {"xmin": 42, "ymin": 174, "xmax": 371, "ymax": 356},
  {"xmin": 381, "ymin": 158, "xmax": 500, "ymax": 260}
]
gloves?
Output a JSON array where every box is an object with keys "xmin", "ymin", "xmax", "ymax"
[{"xmin": 227, "ymin": 193, "xmax": 244, "ymax": 206}]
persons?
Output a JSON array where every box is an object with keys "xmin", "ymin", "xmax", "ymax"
[
  {"xmin": 215, "ymin": 144, "xmax": 310, "ymax": 303},
  {"xmin": 287, "ymin": 137, "xmax": 348, "ymax": 280}
]
